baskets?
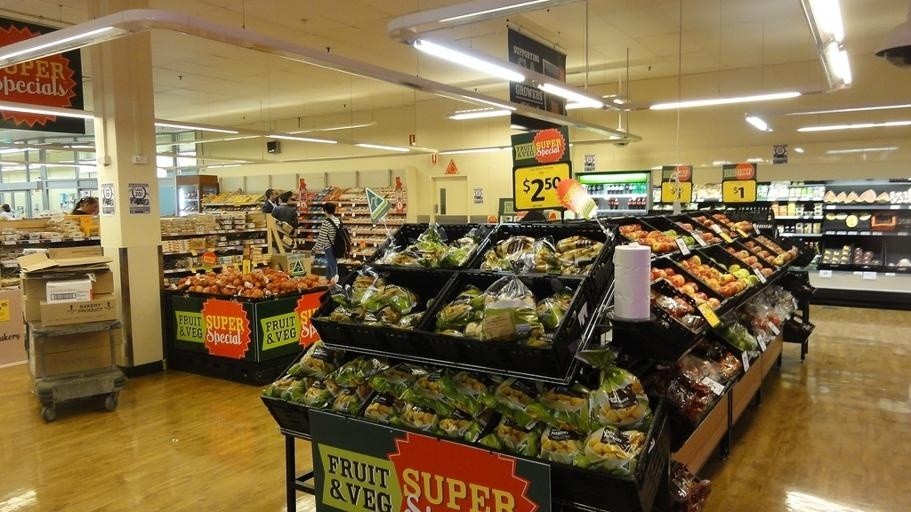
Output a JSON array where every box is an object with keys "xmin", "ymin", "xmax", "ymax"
[
  {"xmin": 783, "ymin": 312, "xmax": 816, "ymax": 345},
  {"xmin": 615, "ymin": 210, "xmax": 797, "ymax": 354},
  {"xmin": 259, "ymin": 345, "xmax": 671, "ymax": 511},
  {"xmin": 781, "ymin": 237, "xmax": 816, "ymax": 267},
  {"xmin": 784, "ymin": 273, "xmax": 818, "ymax": 307},
  {"xmin": 312, "ymin": 222, "xmax": 621, "ymax": 378}
]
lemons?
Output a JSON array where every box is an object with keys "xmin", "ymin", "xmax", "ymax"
[
  {"xmin": 384, "ymin": 237, "xmax": 472, "ymax": 268},
  {"xmin": 481, "ymin": 236, "xmax": 603, "ymax": 275},
  {"xmin": 330, "ymin": 276, "xmax": 434, "ymax": 328},
  {"xmin": 436, "ymin": 289, "xmax": 573, "ymax": 348},
  {"xmin": 273, "ymin": 339, "xmax": 650, "ymax": 472}
]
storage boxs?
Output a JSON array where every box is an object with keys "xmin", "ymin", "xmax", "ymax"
[
  {"xmin": 602, "ymin": 212, "xmax": 804, "ymax": 332},
  {"xmin": 17, "ymin": 245, "xmax": 123, "ymax": 377}
]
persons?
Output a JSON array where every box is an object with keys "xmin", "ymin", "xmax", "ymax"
[
  {"xmin": 310, "ymin": 201, "xmax": 342, "ymax": 281},
  {"xmin": 69, "ymin": 197, "xmax": 99, "ymax": 214},
  {"xmin": 1, "ymin": 203, "xmax": 14, "ymax": 219},
  {"xmin": 272, "ymin": 193, "xmax": 299, "ymax": 240},
  {"xmin": 262, "ymin": 188, "xmax": 278, "ymax": 212}
]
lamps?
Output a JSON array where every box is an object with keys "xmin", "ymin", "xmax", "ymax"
[
  {"xmin": 648, "ymin": 3, "xmax": 803, "ymax": 111},
  {"xmin": 410, "ymin": 3, "xmax": 632, "ymax": 110}
]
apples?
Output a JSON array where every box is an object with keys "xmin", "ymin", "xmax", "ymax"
[
  {"xmin": 619, "ymin": 213, "xmax": 796, "ymax": 309},
  {"xmin": 179, "ymin": 269, "xmax": 328, "ymax": 297}
]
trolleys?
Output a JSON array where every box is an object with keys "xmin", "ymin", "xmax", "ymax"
[{"xmin": 22, "ymin": 315, "xmax": 127, "ymax": 424}]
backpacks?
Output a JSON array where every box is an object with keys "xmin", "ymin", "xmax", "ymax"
[{"xmin": 324, "ymin": 218, "xmax": 350, "ymax": 258}]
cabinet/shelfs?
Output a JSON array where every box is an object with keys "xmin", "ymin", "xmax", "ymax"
[
  {"xmin": 172, "ymin": 174, "xmax": 409, "ymax": 275},
  {"xmin": 767, "ymin": 184, "xmax": 911, "ymax": 272}
]
